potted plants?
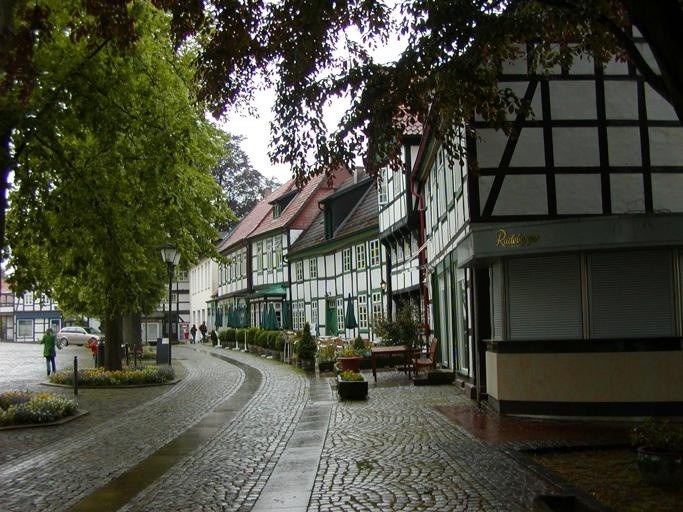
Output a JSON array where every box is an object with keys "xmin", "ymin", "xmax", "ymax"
[
  {"xmin": 219, "ymin": 321, "xmax": 364, "ymax": 373},
  {"xmin": 338, "ymin": 368, "xmax": 368, "ymax": 402},
  {"xmin": 628, "ymin": 417, "xmax": 681, "ymax": 489}
]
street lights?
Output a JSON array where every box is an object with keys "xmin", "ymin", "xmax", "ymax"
[{"xmin": 161, "ymin": 242, "xmax": 182, "ymax": 364}]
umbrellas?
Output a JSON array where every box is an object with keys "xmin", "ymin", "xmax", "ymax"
[
  {"xmin": 345, "ymin": 293, "xmax": 358, "ymax": 339},
  {"xmin": 211, "ymin": 302, "xmax": 293, "ymax": 329}
]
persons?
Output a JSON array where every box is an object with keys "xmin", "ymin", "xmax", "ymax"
[
  {"xmin": 198, "ymin": 320, "xmax": 207, "ymax": 343},
  {"xmin": 37, "ymin": 328, "xmax": 62, "ymax": 376},
  {"xmin": 182, "ymin": 324, "xmax": 189, "ymax": 344},
  {"xmin": 84, "ymin": 336, "xmax": 98, "ymax": 356},
  {"xmin": 189, "ymin": 324, "xmax": 197, "ymax": 344}
]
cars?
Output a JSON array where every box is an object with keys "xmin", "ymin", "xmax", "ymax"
[{"xmin": 57, "ymin": 325, "xmax": 105, "ymax": 348}]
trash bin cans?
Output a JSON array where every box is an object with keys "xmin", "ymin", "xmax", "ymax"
[{"xmin": 156, "ymin": 338, "xmax": 170, "ymax": 365}]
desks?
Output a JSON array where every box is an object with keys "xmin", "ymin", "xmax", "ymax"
[{"xmin": 370, "ymin": 345, "xmax": 412, "ymax": 383}]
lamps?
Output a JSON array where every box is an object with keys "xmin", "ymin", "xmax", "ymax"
[{"xmin": 380, "ymin": 279, "xmax": 388, "ymax": 296}]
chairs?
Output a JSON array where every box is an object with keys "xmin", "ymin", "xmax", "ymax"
[{"xmin": 412, "ymin": 336, "xmax": 439, "ymax": 375}]
